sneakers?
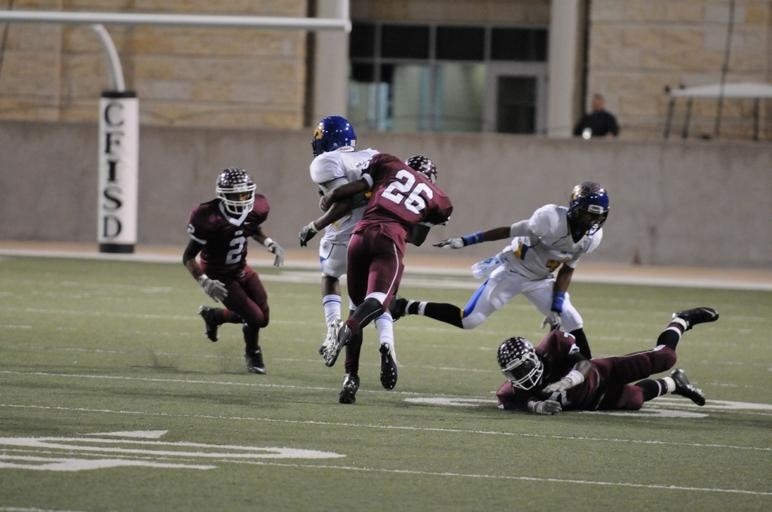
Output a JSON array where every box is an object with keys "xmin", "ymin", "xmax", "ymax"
[
  {"xmin": 378, "ymin": 342, "xmax": 399, "ymax": 391},
  {"xmin": 339, "ymin": 375, "xmax": 359, "ymax": 404},
  {"xmin": 249, "ymin": 359, "xmax": 263, "ymax": 374},
  {"xmin": 671, "ymin": 307, "xmax": 718, "ymax": 328},
  {"xmin": 198, "ymin": 306, "xmax": 216, "ymax": 340},
  {"xmin": 319, "ymin": 319, "xmax": 356, "ymax": 367},
  {"xmin": 668, "ymin": 368, "xmax": 706, "ymax": 405}
]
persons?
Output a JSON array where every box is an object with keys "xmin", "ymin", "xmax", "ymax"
[
  {"xmin": 298, "ymin": 116, "xmax": 398, "ymax": 389},
  {"xmin": 575, "ymin": 95, "xmax": 618, "ymax": 138},
  {"xmin": 496, "ymin": 307, "xmax": 719, "ymax": 415},
  {"xmin": 388, "ymin": 181, "xmax": 610, "ymax": 361},
  {"xmin": 319, "ymin": 153, "xmax": 453, "ymax": 404},
  {"xmin": 183, "ymin": 168, "xmax": 284, "ymax": 374}
]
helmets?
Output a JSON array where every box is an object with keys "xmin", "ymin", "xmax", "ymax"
[
  {"xmin": 216, "ymin": 169, "xmax": 256, "ymax": 216},
  {"xmin": 312, "ymin": 116, "xmax": 357, "ymax": 156},
  {"xmin": 497, "ymin": 338, "xmax": 545, "ymax": 392},
  {"xmin": 570, "ymin": 181, "xmax": 609, "ymax": 234},
  {"xmin": 405, "ymin": 156, "xmax": 439, "ymax": 182}
]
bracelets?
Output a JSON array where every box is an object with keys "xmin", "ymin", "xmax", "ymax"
[
  {"xmin": 463, "ymin": 232, "xmax": 484, "ymax": 245},
  {"xmin": 198, "ymin": 274, "xmax": 209, "ymax": 286},
  {"xmin": 565, "ymin": 370, "xmax": 585, "ymax": 388},
  {"xmin": 551, "ymin": 291, "xmax": 565, "ymax": 312},
  {"xmin": 264, "ymin": 237, "xmax": 275, "ymax": 248}
]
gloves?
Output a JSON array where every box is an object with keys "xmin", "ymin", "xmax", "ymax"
[
  {"xmin": 534, "ymin": 400, "xmax": 562, "ymax": 415},
  {"xmin": 542, "ymin": 376, "xmax": 573, "ymax": 394},
  {"xmin": 299, "ymin": 222, "xmax": 318, "ymax": 246},
  {"xmin": 433, "ymin": 237, "xmax": 466, "ymax": 249},
  {"xmin": 541, "ymin": 309, "xmax": 561, "ymax": 332},
  {"xmin": 197, "ymin": 273, "xmax": 230, "ymax": 302},
  {"xmin": 263, "ymin": 238, "xmax": 284, "ymax": 267}
]
